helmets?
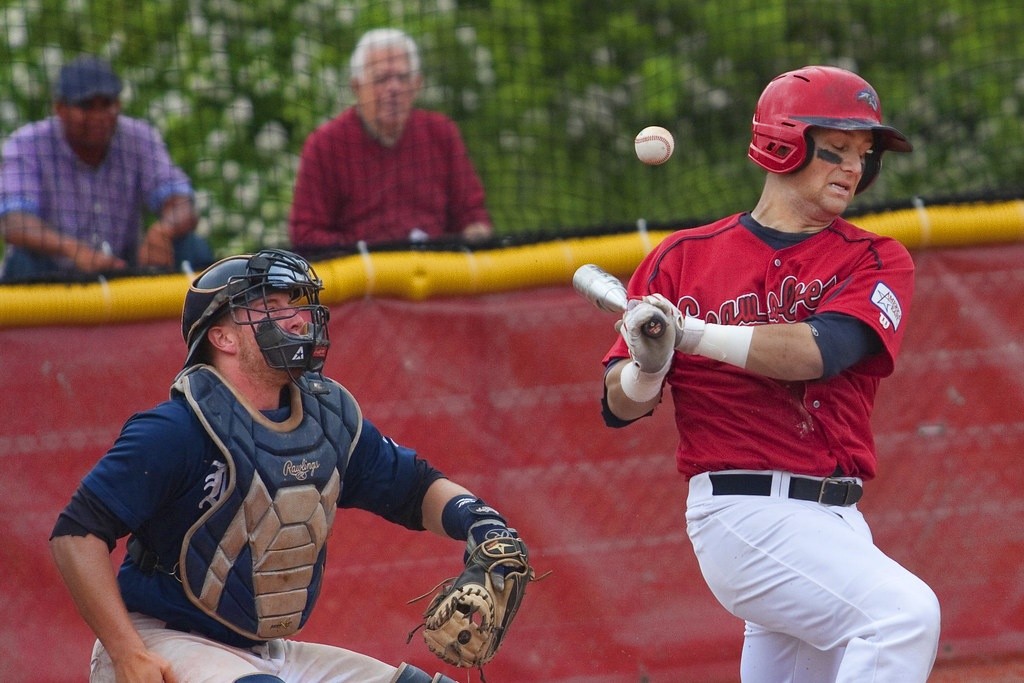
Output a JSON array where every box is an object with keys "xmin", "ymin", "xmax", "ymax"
[
  {"xmin": 181, "ymin": 249, "xmax": 332, "ymax": 370},
  {"xmin": 746, "ymin": 65, "xmax": 917, "ymax": 178}
]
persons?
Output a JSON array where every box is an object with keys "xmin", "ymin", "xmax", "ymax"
[
  {"xmin": 1, "ymin": 61, "xmax": 213, "ymax": 285},
  {"xmin": 290, "ymin": 27, "xmax": 497, "ymax": 246},
  {"xmin": 50, "ymin": 251, "xmax": 536, "ymax": 683},
  {"xmin": 599, "ymin": 64, "xmax": 941, "ymax": 680}
]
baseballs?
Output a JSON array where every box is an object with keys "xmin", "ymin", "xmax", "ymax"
[{"xmin": 633, "ymin": 125, "xmax": 677, "ymax": 165}]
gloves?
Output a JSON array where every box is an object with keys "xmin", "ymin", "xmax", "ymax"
[{"xmin": 618, "ymin": 292, "xmax": 685, "ymax": 374}]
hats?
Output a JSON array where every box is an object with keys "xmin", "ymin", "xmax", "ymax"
[{"xmin": 56, "ymin": 59, "xmax": 124, "ymax": 107}]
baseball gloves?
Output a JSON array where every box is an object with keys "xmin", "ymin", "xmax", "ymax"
[{"xmin": 420, "ymin": 536, "xmax": 536, "ymax": 669}]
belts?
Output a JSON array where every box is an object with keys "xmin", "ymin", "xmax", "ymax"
[{"xmin": 710, "ymin": 475, "xmax": 864, "ymax": 508}]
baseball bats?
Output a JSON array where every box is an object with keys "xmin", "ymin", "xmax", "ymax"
[{"xmin": 571, "ymin": 263, "xmax": 668, "ymax": 341}]
